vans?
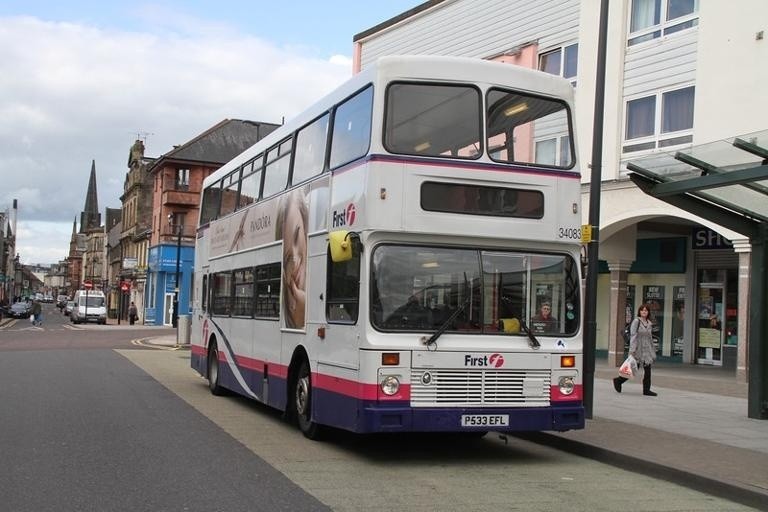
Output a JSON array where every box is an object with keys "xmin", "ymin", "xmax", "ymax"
[{"xmin": 72, "ymin": 289, "xmax": 107, "ymax": 324}]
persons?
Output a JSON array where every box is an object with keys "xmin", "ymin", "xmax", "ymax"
[
  {"xmin": 128, "ymin": 302, "xmax": 137, "ymax": 325},
  {"xmin": 531, "ymin": 301, "xmax": 557, "ymax": 332},
  {"xmin": 275, "ymin": 189, "xmax": 309, "ymax": 330},
  {"xmin": 613, "ymin": 304, "xmax": 657, "ymax": 395},
  {"xmin": 28, "ymin": 300, "xmax": 42, "ymax": 327}
]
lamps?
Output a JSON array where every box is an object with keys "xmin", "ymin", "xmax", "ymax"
[
  {"xmin": 503, "ymin": 47, "xmax": 520, "ymax": 56},
  {"xmin": 503, "ymin": 102, "xmax": 530, "ymax": 116},
  {"xmin": 414, "ymin": 142, "xmax": 432, "ymax": 152}
]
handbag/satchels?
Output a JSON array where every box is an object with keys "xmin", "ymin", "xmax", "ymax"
[{"xmin": 621, "ymin": 318, "xmax": 640, "ymax": 345}]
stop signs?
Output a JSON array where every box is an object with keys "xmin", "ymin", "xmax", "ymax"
[{"xmin": 84, "ymin": 280, "xmax": 93, "ymax": 290}]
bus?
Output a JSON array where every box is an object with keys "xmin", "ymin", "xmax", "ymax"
[{"xmin": 189, "ymin": 55, "xmax": 586, "ymax": 440}]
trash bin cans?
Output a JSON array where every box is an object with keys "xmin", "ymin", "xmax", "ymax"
[{"xmin": 177, "ymin": 314, "xmax": 191, "ymax": 344}]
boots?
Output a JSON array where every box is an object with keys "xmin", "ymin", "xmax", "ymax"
[
  {"xmin": 613, "ymin": 376, "xmax": 629, "ymax": 393},
  {"xmin": 643, "ymin": 379, "xmax": 657, "ymax": 396}
]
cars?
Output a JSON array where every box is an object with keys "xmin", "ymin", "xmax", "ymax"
[
  {"xmin": 29, "ymin": 292, "xmax": 54, "ymax": 304},
  {"xmin": 6, "ymin": 300, "xmax": 41, "ymax": 318},
  {"xmin": 56, "ymin": 295, "xmax": 75, "ymax": 316}
]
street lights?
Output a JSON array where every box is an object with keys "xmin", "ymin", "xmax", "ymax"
[{"xmin": 167, "ymin": 212, "xmax": 183, "ymax": 328}]
